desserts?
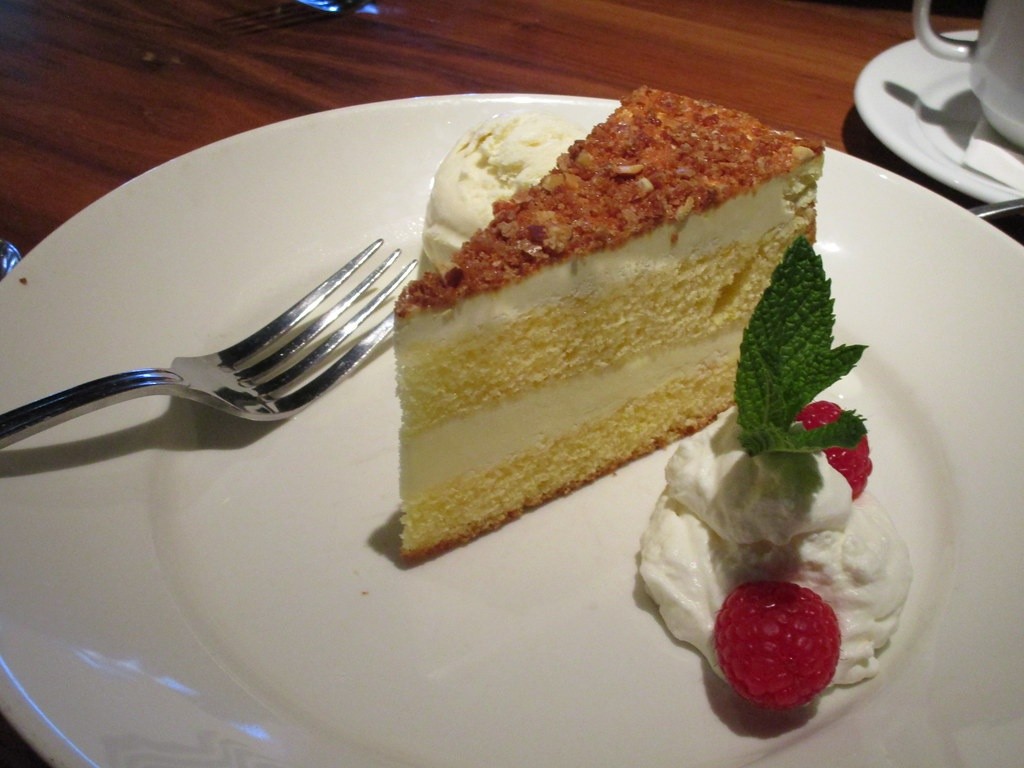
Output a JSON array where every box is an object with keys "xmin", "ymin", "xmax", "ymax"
[
  {"xmin": 634, "ymin": 236, "xmax": 916, "ymax": 715},
  {"xmin": 391, "ymin": 81, "xmax": 825, "ymax": 571}
]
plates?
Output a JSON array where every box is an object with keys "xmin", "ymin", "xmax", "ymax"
[
  {"xmin": 3, "ymin": 92, "xmax": 1023, "ymax": 768},
  {"xmin": 854, "ymin": 30, "xmax": 1024, "ymax": 205}
]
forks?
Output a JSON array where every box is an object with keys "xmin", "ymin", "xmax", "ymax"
[
  {"xmin": 217, "ymin": 0, "xmax": 373, "ymax": 35},
  {"xmin": 1, "ymin": 239, "xmax": 419, "ymax": 447}
]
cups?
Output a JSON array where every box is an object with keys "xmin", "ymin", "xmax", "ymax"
[{"xmin": 912, "ymin": 0, "xmax": 1024, "ymax": 148}]
